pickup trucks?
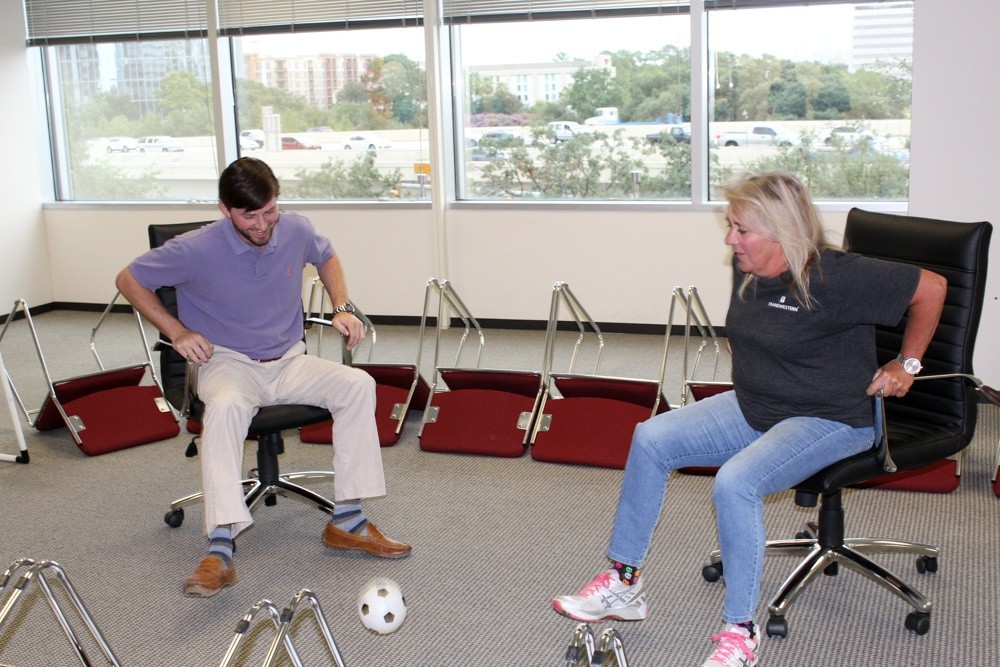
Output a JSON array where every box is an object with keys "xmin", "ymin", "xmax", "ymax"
[
  {"xmin": 136, "ymin": 136, "xmax": 185, "ymax": 153},
  {"xmin": 716, "ymin": 125, "xmax": 796, "ymax": 147},
  {"xmin": 646, "ymin": 126, "xmax": 692, "ymax": 146}
]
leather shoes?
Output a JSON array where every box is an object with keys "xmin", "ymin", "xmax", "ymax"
[
  {"xmin": 322, "ymin": 521, "xmax": 412, "ymax": 558},
  {"xmin": 184, "ymin": 556, "xmax": 236, "ymax": 595}
]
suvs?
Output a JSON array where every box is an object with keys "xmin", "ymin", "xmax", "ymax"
[
  {"xmin": 545, "ymin": 121, "xmax": 591, "ymax": 144},
  {"xmin": 239, "ymin": 129, "xmax": 266, "ymax": 150}
]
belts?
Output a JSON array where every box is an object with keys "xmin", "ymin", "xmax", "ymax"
[{"xmin": 251, "ymin": 356, "xmax": 282, "ymax": 362}]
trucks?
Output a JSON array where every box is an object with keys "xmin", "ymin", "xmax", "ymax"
[{"xmin": 582, "ymin": 107, "xmax": 683, "ymax": 125}]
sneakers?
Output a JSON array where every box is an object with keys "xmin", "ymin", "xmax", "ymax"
[
  {"xmin": 551, "ymin": 569, "xmax": 646, "ymax": 621},
  {"xmin": 700, "ymin": 623, "xmax": 761, "ymax": 667}
]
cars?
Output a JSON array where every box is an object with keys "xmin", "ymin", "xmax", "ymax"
[
  {"xmin": 468, "ymin": 138, "xmax": 504, "ymax": 161},
  {"xmin": 106, "ymin": 136, "xmax": 138, "ymax": 153},
  {"xmin": 817, "ymin": 124, "xmax": 875, "ymax": 146},
  {"xmin": 479, "ymin": 130, "xmax": 529, "ymax": 147},
  {"xmin": 339, "ymin": 134, "xmax": 393, "ymax": 150},
  {"xmin": 281, "ymin": 135, "xmax": 321, "ymax": 150}
]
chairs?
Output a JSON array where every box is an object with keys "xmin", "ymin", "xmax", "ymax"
[
  {"xmin": 0, "ymin": 555, "xmax": 631, "ymax": 666},
  {"xmin": 0, "ymin": 286, "xmax": 179, "ymax": 455},
  {"xmin": 684, "ymin": 286, "xmax": 741, "ymax": 476},
  {"xmin": 187, "ymin": 277, "xmax": 321, "ymax": 436},
  {"xmin": 302, "ymin": 276, "xmax": 469, "ymax": 446},
  {"xmin": 532, "ymin": 284, "xmax": 706, "ymax": 471},
  {"xmin": 419, "ymin": 279, "xmax": 584, "ymax": 459},
  {"xmin": 702, "ymin": 207, "xmax": 1000, "ymax": 638},
  {"xmin": 148, "ymin": 220, "xmax": 342, "ymax": 528}
]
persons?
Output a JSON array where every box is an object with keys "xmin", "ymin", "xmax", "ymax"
[
  {"xmin": 115, "ymin": 155, "xmax": 412, "ymax": 598},
  {"xmin": 553, "ymin": 171, "xmax": 948, "ymax": 667}
]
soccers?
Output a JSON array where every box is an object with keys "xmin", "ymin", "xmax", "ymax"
[{"xmin": 357, "ymin": 584, "xmax": 409, "ymax": 636}]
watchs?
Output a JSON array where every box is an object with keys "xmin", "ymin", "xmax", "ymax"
[
  {"xmin": 896, "ymin": 354, "xmax": 922, "ymax": 376},
  {"xmin": 333, "ymin": 302, "xmax": 356, "ymax": 314}
]
